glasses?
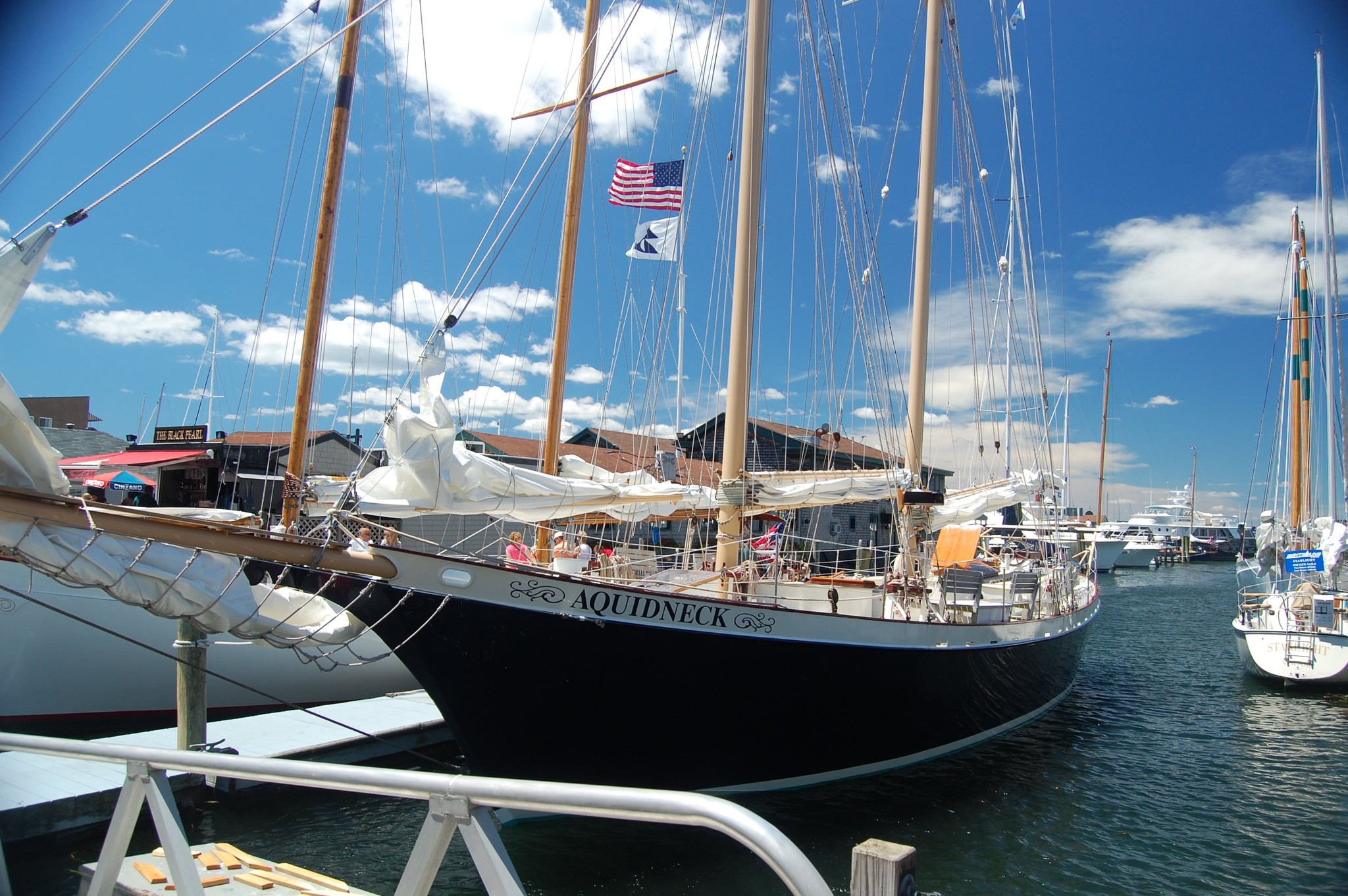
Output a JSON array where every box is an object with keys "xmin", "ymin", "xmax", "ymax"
[
  {"xmin": 384, "ymin": 534, "xmax": 389, "ymax": 536},
  {"xmin": 361, "ymin": 533, "xmax": 371, "ymax": 536}
]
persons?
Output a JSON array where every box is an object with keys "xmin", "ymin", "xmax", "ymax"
[
  {"xmin": 574, "ymin": 530, "xmax": 592, "ymax": 583},
  {"xmin": 379, "ymin": 526, "xmax": 402, "ymax": 549},
  {"xmin": 506, "ymin": 532, "xmax": 539, "ymax": 570},
  {"xmin": 82, "ymin": 484, "xmax": 159, "ymax": 507},
  {"xmin": 553, "ymin": 532, "xmax": 573, "ymax": 579},
  {"xmin": 593, "ymin": 544, "xmax": 615, "ymax": 577},
  {"xmin": 660, "ymin": 555, "xmax": 674, "ymax": 570},
  {"xmin": 349, "ymin": 528, "xmax": 374, "ymax": 549},
  {"xmin": 1085, "ymin": 519, "xmax": 1092, "ymax": 528},
  {"xmin": 736, "ymin": 533, "xmax": 757, "ymax": 602}
]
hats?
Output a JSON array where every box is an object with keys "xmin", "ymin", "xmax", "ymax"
[{"xmin": 553, "ymin": 532, "xmax": 565, "ymax": 540}]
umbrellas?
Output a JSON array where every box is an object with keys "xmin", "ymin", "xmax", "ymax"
[{"xmin": 82, "ymin": 470, "xmax": 156, "ymax": 503}]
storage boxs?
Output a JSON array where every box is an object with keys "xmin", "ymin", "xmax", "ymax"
[{"xmin": 1313, "ymin": 594, "xmax": 1333, "ymax": 628}]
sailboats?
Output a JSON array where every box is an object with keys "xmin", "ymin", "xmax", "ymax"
[{"xmin": 3, "ymin": 0, "xmax": 1346, "ymax": 896}]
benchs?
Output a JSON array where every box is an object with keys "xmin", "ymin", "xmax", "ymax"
[
  {"xmin": 980, "ymin": 573, "xmax": 1039, "ymax": 621},
  {"xmin": 889, "ymin": 567, "xmax": 986, "ymax": 623}
]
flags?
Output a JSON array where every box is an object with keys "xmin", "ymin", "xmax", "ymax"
[
  {"xmin": 752, "ymin": 511, "xmax": 790, "ymax": 563},
  {"xmin": 625, "ymin": 214, "xmax": 680, "ymax": 262},
  {"xmin": 606, "ymin": 157, "xmax": 685, "ymax": 213}
]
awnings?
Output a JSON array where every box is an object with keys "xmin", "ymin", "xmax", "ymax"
[{"xmin": 58, "ymin": 450, "xmax": 215, "ymax": 471}]
